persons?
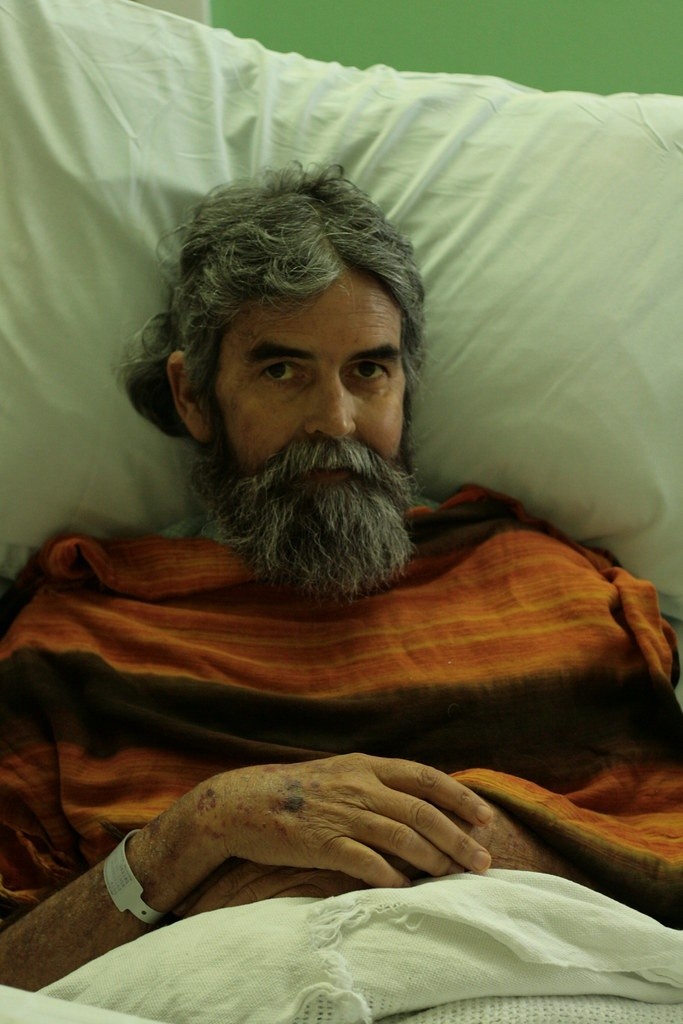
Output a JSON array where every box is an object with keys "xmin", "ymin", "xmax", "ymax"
[{"xmin": 1, "ymin": 156, "xmax": 683, "ymax": 995}]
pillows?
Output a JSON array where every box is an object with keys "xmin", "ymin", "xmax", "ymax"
[{"xmin": 3, "ymin": 0, "xmax": 681, "ymax": 633}]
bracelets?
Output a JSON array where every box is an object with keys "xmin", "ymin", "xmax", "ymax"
[{"xmin": 103, "ymin": 830, "xmax": 162, "ymax": 926}]
